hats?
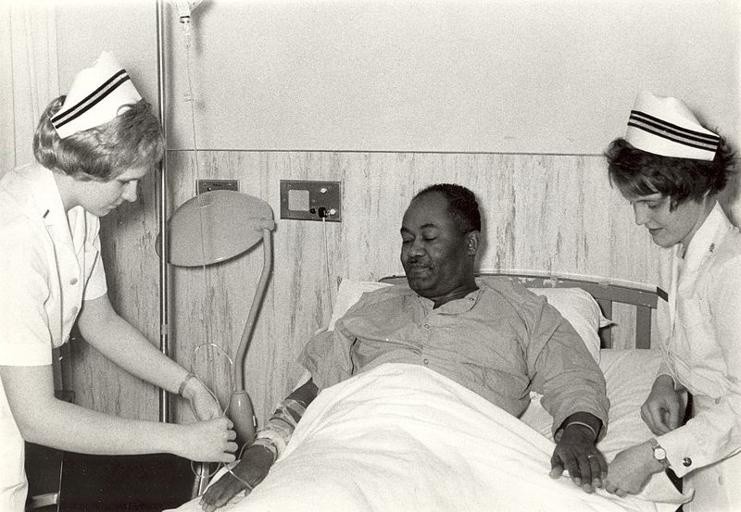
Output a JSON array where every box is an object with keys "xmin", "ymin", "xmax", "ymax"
[
  {"xmin": 50, "ymin": 50, "xmax": 143, "ymax": 140},
  {"xmin": 624, "ymin": 91, "xmax": 720, "ymax": 162}
]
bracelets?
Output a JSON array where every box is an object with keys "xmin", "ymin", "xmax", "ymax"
[
  {"xmin": 176, "ymin": 373, "xmax": 196, "ymax": 396},
  {"xmin": 563, "ymin": 420, "xmax": 597, "ymax": 438}
]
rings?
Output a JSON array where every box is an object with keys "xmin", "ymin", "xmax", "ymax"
[{"xmin": 587, "ymin": 454, "xmax": 597, "ymax": 459}]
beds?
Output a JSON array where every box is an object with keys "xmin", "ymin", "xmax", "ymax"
[{"xmin": 297, "ymin": 273, "xmax": 680, "ymax": 512}]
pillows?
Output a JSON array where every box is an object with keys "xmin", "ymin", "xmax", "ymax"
[{"xmin": 294, "ymin": 277, "xmax": 619, "ymax": 447}]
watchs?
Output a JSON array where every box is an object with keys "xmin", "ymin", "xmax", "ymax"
[{"xmin": 649, "ymin": 436, "xmax": 670, "ymax": 471}]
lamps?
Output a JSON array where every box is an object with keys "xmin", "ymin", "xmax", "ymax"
[{"xmin": 154, "ymin": 191, "xmax": 276, "ymax": 444}]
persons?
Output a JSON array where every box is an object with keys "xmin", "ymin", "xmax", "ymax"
[
  {"xmin": 2, "ymin": 50, "xmax": 240, "ymax": 512},
  {"xmin": 603, "ymin": 88, "xmax": 741, "ymax": 512},
  {"xmin": 200, "ymin": 184, "xmax": 611, "ymax": 512}
]
